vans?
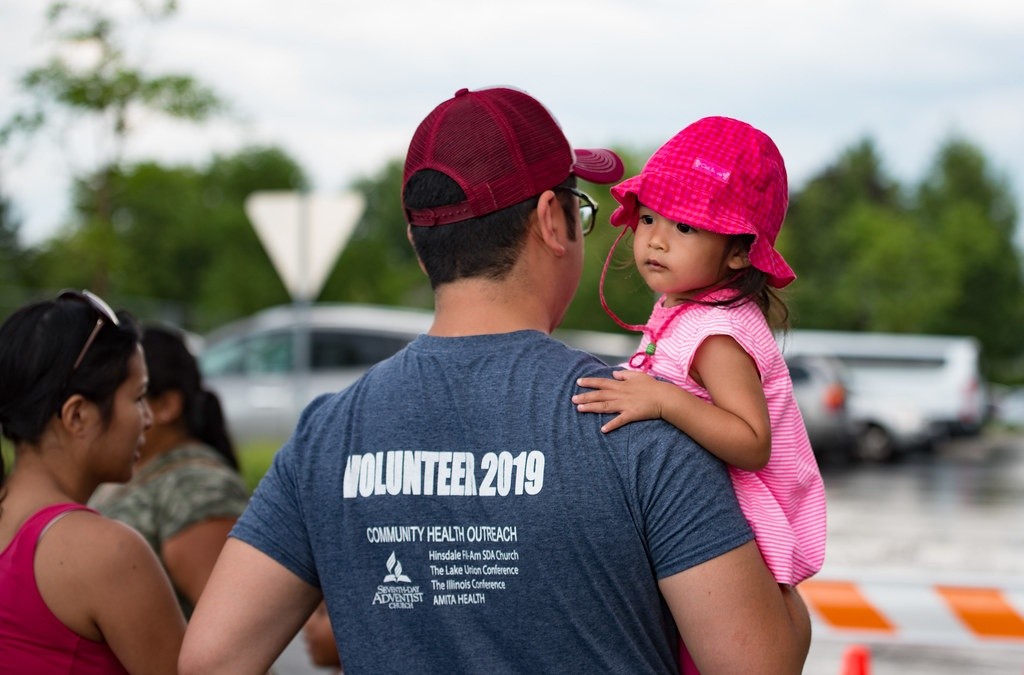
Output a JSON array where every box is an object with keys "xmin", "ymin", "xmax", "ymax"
[{"xmin": 780, "ymin": 330, "xmax": 987, "ymax": 462}]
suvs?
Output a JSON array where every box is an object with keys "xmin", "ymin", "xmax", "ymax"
[{"xmin": 789, "ymin": 358, "xmax": 861, "ymax": 467}]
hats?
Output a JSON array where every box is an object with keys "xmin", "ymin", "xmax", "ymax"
[
  {"xmin": 600, "ymin": 116, "xmax": 797, "ymax": 372},
  {"xmin": 400, "ymin": 86, "xmax": 625, "ymax": 228}
]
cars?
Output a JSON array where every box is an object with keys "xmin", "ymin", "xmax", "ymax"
[{"xmin": 197, "ymin": 307, "xmax": 431, "ymax": 443}]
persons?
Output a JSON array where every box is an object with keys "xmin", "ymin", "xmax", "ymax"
[
  {"xmin": 571, "ymin": 116, "xmax": 827, "ymax": 675},
  {"xmin": 176, "ymin": 87, "xmax": 811, "ymax": 675},
  {"xmin": 0, "ymin": 288, "xmax": 346, "ymax": 675}
]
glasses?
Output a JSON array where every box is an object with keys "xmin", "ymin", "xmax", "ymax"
[
  {"xmin": 70, "ymin": 289, "xmax": 118, "ymax": 371},
  {"xmin": 548, "ymin": 184, "xmax": 600, "ymax": 236}
]
